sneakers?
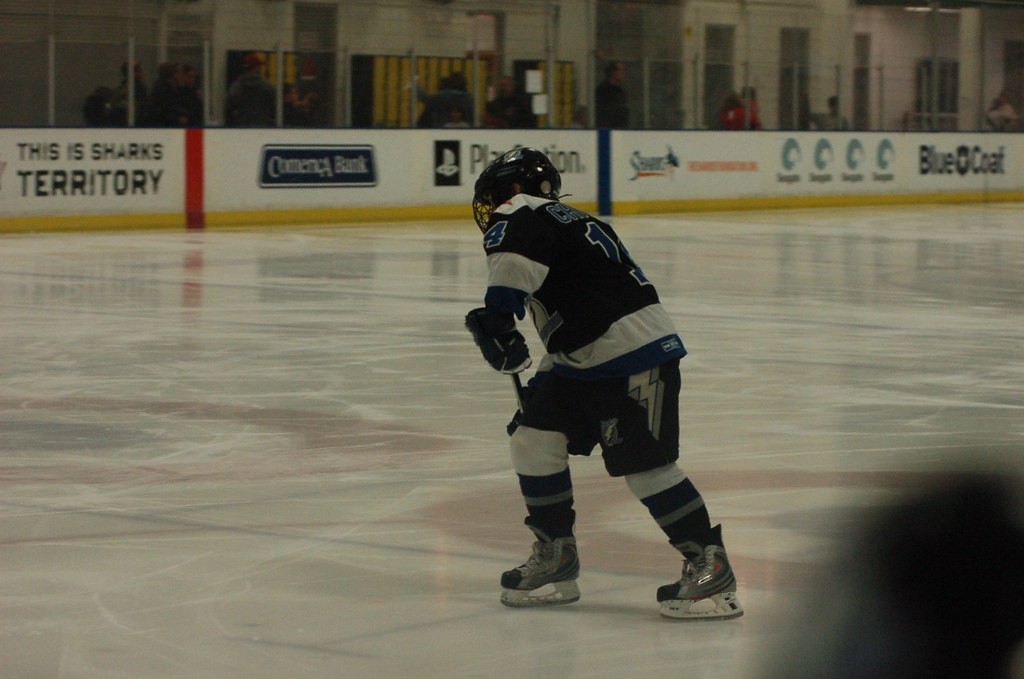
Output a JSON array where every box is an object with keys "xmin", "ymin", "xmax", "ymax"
[
  {"xmin": 657, "ymin": 523, "xmax": 743, "ymax": 619},
  {"xmin": 500, "ymin": 510, "xmax": 580, "ymax": 607}
]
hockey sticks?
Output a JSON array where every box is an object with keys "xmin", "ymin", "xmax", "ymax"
[{"xmin": 509, "ymin": 371, "xmax": 525, "ymax": 399}]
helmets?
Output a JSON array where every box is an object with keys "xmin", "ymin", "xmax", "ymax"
[{"xmin": 472, "ymin": 146, "xmax": 561, "ymax": 234}]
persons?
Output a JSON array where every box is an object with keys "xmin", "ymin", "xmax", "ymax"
[
  {"xmin": 720, "ymin": 87, "xmax": 760, "ymax": 128},
  {"xmin": 801, "ymin": 471, "xmax": 1024, "ymax": 679},
  {"xmin": 809, "ymin": 95, "xmax": 849, "ymax": 131},
  {"xmin": 560, "ymin": 76, "xmax": 589, "ymax": 128},
  {"xmin": 479, "ymin": 75, "xmax": 537, "ymax": 128},
  {"xmin": 982, "ymin": 92, "xmax": 1018, "ymax": 131},
  {"xmin": 412, "ymin": 71, "xmax": 474, "ymax": 128},
  {"xmin": 464, "ymin": 146, "xmax": 744, "ymax": 621},
  {"xmin": 84, "ymin": 54, "xmax": 325, "ymax": 128},
  {"xmin": 595, "ymin": 61, "xmax": 631, "ymax": 127}
]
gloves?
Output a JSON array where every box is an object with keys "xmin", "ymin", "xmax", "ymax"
[{"xmin": 465, "ymin": 307, "xmax": 532, "ymax": 375}]
